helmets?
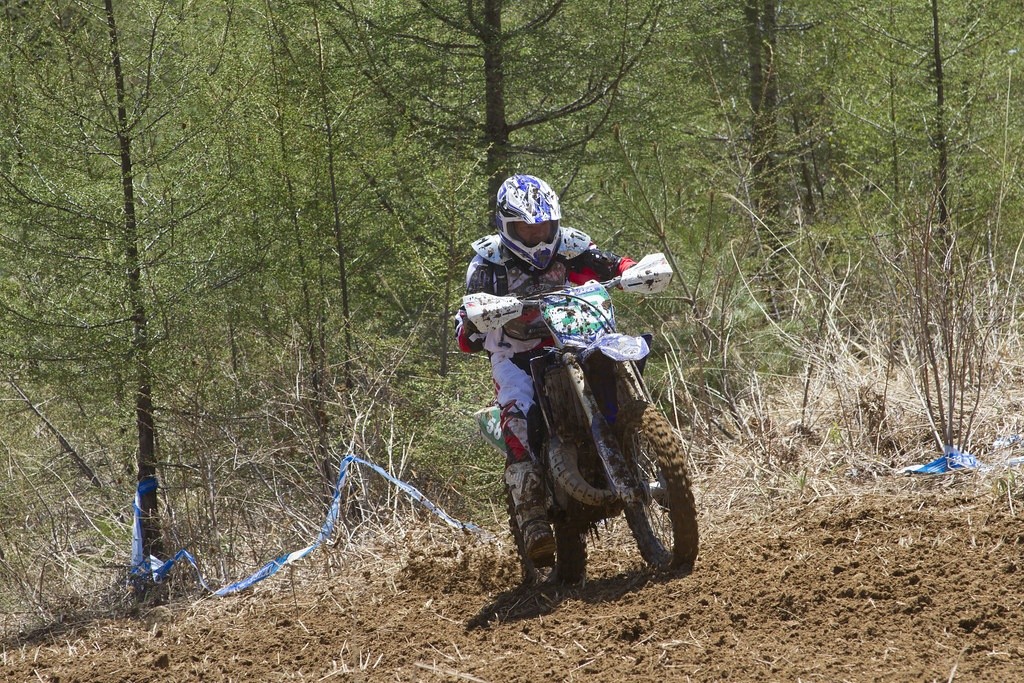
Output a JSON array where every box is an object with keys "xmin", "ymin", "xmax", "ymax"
[{"xmin": 495, "ymin": 174, "xmax": 561, "ymax": 271}]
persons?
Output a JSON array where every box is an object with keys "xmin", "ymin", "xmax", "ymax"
[{"xmin": 455, "ymin": 173, "xmax": 639, "ymax": 565}]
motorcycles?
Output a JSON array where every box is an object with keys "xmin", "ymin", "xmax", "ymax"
[{"xmin": 458, "ymin": 250, "xmax": 697, "ymax": 593}]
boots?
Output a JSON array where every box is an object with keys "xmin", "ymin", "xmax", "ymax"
[{"xmin": 504, "ymin": 462, "xmax": 556, "ymax": 567}]
